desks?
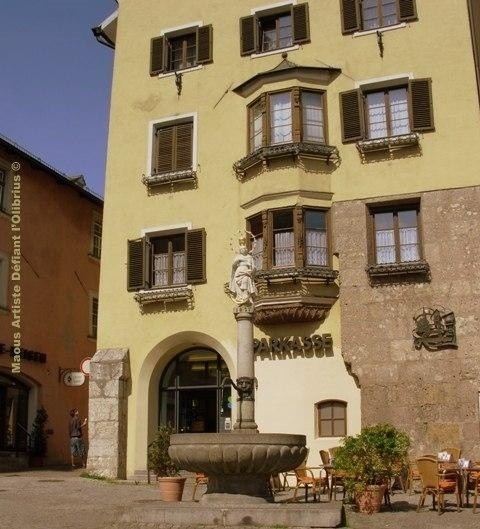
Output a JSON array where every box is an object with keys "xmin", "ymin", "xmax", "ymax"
[{"xmin": 437, "ymin": 462, "xmax": 480, "ymax": 515}]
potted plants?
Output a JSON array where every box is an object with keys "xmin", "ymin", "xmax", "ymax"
[
  {"xmin": 28, "ymin": 408, "xmax": 55, "ymax": 468},
  {"xmin": 146, "ymin": 420, "xmax": 187, "ymax": 503},
  {"xmin": 330, "ymin": 423, "xmax": 412, "ymax": 514}
]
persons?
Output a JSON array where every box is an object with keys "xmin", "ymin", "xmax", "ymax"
[
  {"xmin": 67, "ymin": 408, "xmax": 88, "ymax": 468},
  {"xmin": 227, "ymin": 231, "xmax": 260, "ymax": 304}
]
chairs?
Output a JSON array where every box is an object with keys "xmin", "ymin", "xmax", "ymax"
[
  {"xmin": 190, "ymin": 471, "xmax": 209, "ymax": 502},
  {"xmin": 415, "ymin": 447, "xmax": 466, "ymax": 516},
  {"xmin": 280, "ymin": 449, "xmax": 347, "ymax": 503}
]
